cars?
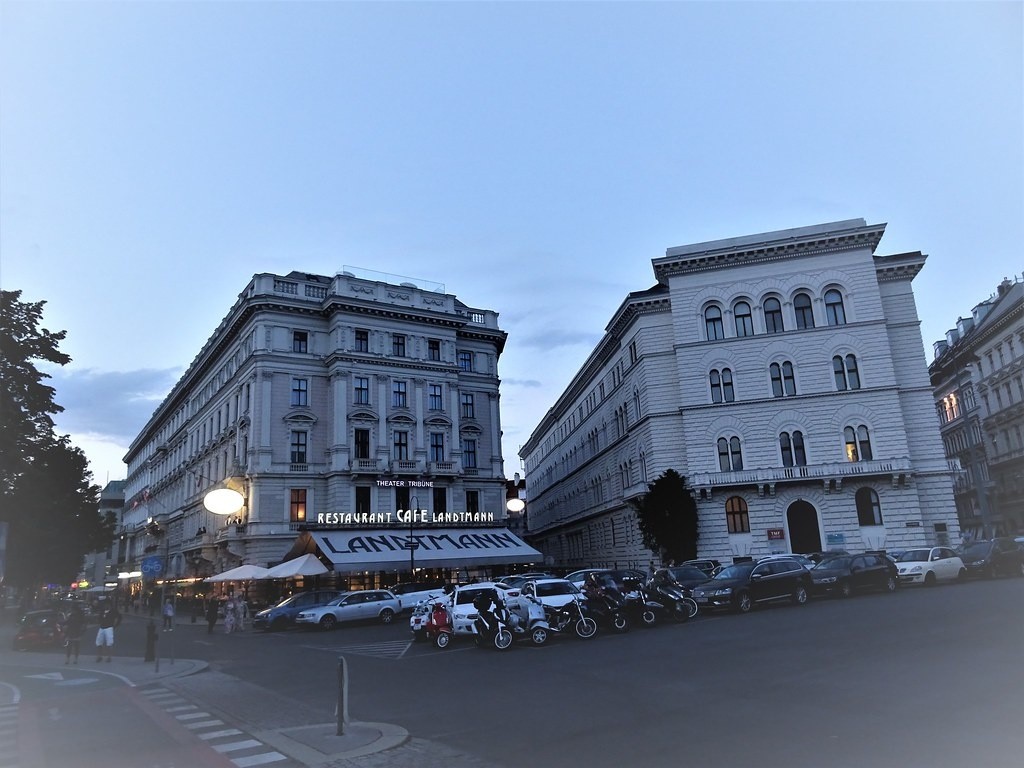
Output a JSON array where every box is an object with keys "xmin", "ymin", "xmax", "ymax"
[
  {"xmin": 11, "ymin": 601, "xmax": 114, "ymax": 651},
  {"xmin": 251, "ymin": 534, "xmax": 1023, "ymax": 639}
]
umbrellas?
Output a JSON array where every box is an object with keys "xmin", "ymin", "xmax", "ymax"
[
  {"xmin": 252, "ymin": 553, "xmax": 329, "ymax": 596},
  {"xmin": 83, "ymin": 586, "xmax": 114, "ymax": 595},
  {"xmin": 203, "ymin": 563, "xmax": 273, "ymax": 618}
]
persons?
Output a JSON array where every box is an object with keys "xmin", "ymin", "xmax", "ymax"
[
  {"xmin": 222, "ymin": 597, "xmax": 235, "ymax": 635},
  {"xmin": 234, "ymin": 595, "xmax": 250, "ymax": 633},
  {"xmin": 443, "ymin": 578, "xmax": 455, "ymax": 595},
  {"xmin": 667, "ymin": 560, "xmax": 676, "ymax": 568},
  {"xmin": 648, "ymin": 561, "xmax": 657, "ymax": 572},
  {"xmin": 205, "ymin": 596, "xmax": 218, "ymax": 635},
  {"xmin": 162, "ymin": 599, "xmax": 174, "ymax": 632},
  {"xmin": 471, "ymin": 576, "xmax": 478, "ymax": 584},
  {"xmin": 95, "ymin": 595, "xmax": 123, "ymax": 662},
  {"xmin": 134, "ymin": 598, "xmax": 140, "ymax": 615},
  {"xmin": 61, "ymin": 604, "xmax": 88, "ymax": 664}
]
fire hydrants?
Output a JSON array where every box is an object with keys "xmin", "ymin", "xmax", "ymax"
[{"xmin": 144, "ymin": 622, "xmax": 160, "ymax": 662}]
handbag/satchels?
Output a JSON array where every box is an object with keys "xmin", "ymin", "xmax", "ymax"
[{"xmin": 167, "ymin": 610, "xmax": 174, "ymax": 616}]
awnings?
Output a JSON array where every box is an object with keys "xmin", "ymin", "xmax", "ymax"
[{"xmin": 282, "ymin": 527, "xmax": 544, "ymax": 591}]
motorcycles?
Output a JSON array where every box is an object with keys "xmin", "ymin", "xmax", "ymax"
[{"xmin": 408, "ymin": 567, "xmax": 700, "ymax": 651}]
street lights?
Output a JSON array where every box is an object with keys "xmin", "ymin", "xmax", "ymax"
[{"xmin": 409, "ymin": 495, "xmax": 422, "ymax": 579}]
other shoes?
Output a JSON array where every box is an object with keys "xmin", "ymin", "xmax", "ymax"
[
  {"xmin": 163, "ymin": 629, "xmax": 173, "ymax": 631},
  {"xmin": 65, "ymin": 661, "xmax": 77, "ymax": 664},
  {"xmin": 95, "ymin": 657, "xmax": 112, "ymax": 662}
]
